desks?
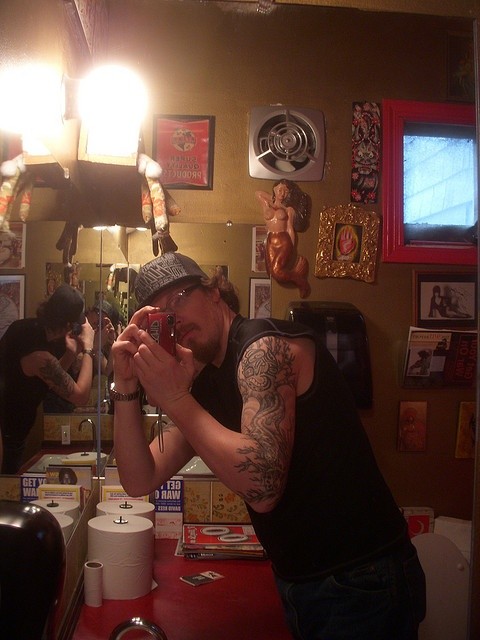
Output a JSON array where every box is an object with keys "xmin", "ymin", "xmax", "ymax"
[{"xmin": 71, "ymin": 532, "xmax": 295, "ymax": 638}]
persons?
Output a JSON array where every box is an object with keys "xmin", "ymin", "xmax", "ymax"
[
  {"xmin": 253, "ymin": 179, "xmax": 312, "ymax": 299},
  {"xmin": 84, "ymin": 301, "xmax": 114, "ymax": 331},
  {"xmin": 0, "ymin": 283, "xmax": 91, "ymax": 475},
  {"xmin": 339, "ymin": 227, "xmax": 355, "ymax": 255},
  {"xmin": 110, "ymin": 252, "xmax": 427, "ymax": 637},
  {"xmin": 42, "ymin": 299, "xmax": 118, "ymax": 414},
  {"xmin": 428, "ymin": 286, "xmax": 472, "ymax": 318},
  {"xmin": 56, "ymin": 469, "xmax": 78, "ymax": 484},
  {"xmin": 214, "ymin": 264, "xmax": 224, "ymax": 283},
  {"xmin": 400, "ymin": 405, "xmax": 420, "ymax": 452}
]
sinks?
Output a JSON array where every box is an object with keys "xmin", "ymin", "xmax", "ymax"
[{"xmin": 39, "ymin": 454, "xmax": 69, "ymax": 470}]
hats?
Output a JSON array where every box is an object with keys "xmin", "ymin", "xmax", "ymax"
[
  {"xmin": 135, "ymin": 252, "xmax": 212, "ymax": 311},
  {"xmin": 48, "ymin": 282, "xmax": 85, "ymax": 323},
  {"xmin": 93, "ymin": 300, "xmax": 113, "ymax": 319}
]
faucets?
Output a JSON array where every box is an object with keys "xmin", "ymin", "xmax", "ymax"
[{"xmin": 78, "ymin": 418, "xmax": 97, "ymax": 451}]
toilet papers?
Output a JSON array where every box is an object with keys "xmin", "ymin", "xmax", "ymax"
[
  {"xmin": 31, "ymin": 499, "xmax": 85, "ymax": 519},
  {"xmin": 86, "ymin": 514, "xmax": 159, "ymax": 599},
  {"xmin": 55, "ymin": 514, "xmax": 75, "ymax": 542},
  {"xmin": 94, "ymin": 499, "xmax": 155, "ymax": 514}
]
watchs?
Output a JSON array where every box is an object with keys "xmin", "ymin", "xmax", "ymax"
[
  {"xmin": 107, "ymin": 382, "xmax": 142, "ymax": 402},
  {"xmin": 82, "ymin": 348, "xmax": 97, "ymax": 359},
  {"xmin": 108, "ymin": 336, "xmax": 114, "ymax": 340}
]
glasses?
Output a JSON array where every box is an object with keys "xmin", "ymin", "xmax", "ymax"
[{"xmin": 159, "ymin": 283, "xmax": 205, "ymax": 312}]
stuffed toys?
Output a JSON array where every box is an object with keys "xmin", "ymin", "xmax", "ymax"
[
  {"xmin": 1, "ymin": 152, "xmax": 34, "ymax": 265},
  {"xmin": 138, "ymin": 152, "xmax": 181, "ymax": 256}
]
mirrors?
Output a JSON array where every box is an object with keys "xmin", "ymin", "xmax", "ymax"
[{"xmin": 2, "ymin": 219, "xmax": 139, "ymax": 638}]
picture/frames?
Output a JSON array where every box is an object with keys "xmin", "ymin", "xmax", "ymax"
[
  {"xmin": 150, "ymin": 112, "xmax": 216, "ymax": 192},
  {"xmin": 314, "ymin": 202, "xmax": 382, "ymax": 284},
  {"xmin": 0, "ymin": 273, "xmax": 25, "ymax": 336},
  {"xmin": 410, "ymin": 268, "xmax": 480, "ymax": 333},
  {"xmin": 248, "ymin": 274, "xmax": 272, "ymax": 322},
  {"xmin": 397, "ymin": 399, "xmax": 430, "ymax": 455}
]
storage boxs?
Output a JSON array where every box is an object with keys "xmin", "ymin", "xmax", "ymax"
[{"xmin": 38, "ymin": 413, "xmax": 114, "ymax": 445}]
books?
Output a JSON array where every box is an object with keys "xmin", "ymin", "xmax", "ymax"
[
  {"xmin": 180, "ymin": 525, "xmax": 268, "ymax": 560},
  {"xmin": 401, "ymin": 326, "xmax": 477, "ymax": 390},
  {"xmin": 105, "ymin": 467, "xmax": 121, "ymax": 486},
  {"xmin": 148, "ymin": 476, "xmax": 184, "ymax": 540},
  {"xmin": 20, "ymin": 473, "xmax": 49, "ymax": 503},
  {"xmin": 38, "ymin": 485, "xmax": 83, "ymax": 507},
  {"xmin": 102, "ymin": 486, "xmax": 151, "ymax": 504},
  {"xmin": 49, "ymin": 467, "xmax": 93, "ymax": 493}
]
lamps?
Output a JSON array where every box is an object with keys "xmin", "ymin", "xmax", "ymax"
[{"xmin": 4, "ymin": 53, "xmax": 151, "ymax": 238}]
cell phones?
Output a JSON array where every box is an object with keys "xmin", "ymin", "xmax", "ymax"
[{"xmin": 148, "ymin": 311, "xmax": 176, "ymax": 358}]
